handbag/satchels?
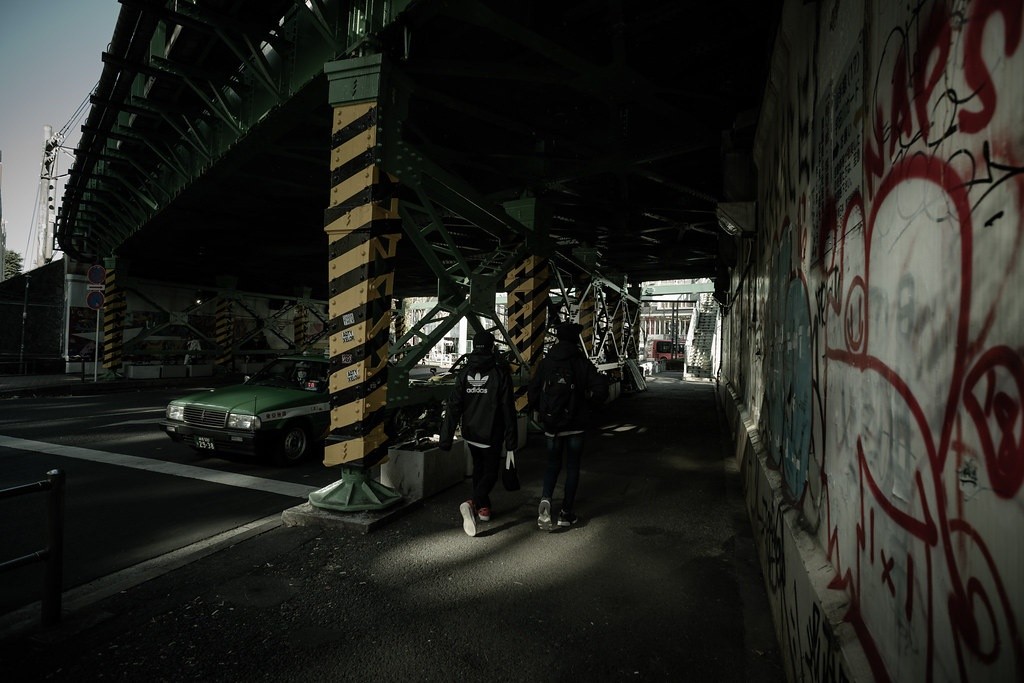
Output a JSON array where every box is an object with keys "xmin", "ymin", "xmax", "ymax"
[{"xmin": 503, "ymin": 451, "xmax": 521, "ymax": 491}]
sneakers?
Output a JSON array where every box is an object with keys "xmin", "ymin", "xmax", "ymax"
[
  {"xmin": 538, "ymin": 496, "xmax": 552, "ymax": 531},
  {"xmin": 460, "ymin": 500, "xmax": 477, "ymax": 536},
  {"xmin": 478, "ymin": 508, "xmax": 491, "ymax": 521},
  {"xmin": 557, "ymin": 515, "xmax": 578, "ymax": 526}
]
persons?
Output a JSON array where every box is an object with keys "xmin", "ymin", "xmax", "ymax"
[
  {"xmin": 528, "ymin": 323, "xmax": 611, "ymax": 531},
  {"xmin": 439, "ymin": 330, "xmax": 519, "ymax": 538},
  {"xmin": 187, "ymin": 336, "xmax": 201, "ymax": 364}
]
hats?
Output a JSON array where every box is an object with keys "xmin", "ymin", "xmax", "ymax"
[
  {"xmin": 557, "ymin": 322, "xmax": 584, "ymax": 340},
  {"xmin": 473, "ymin": 331, "xmax": 495, "ymax": 350}
]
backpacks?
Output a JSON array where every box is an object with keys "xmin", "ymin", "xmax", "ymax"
[{"xmin": 544, "ymin": 357, "xmax": 591, "ymax": 431}]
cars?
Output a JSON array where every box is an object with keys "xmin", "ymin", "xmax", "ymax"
[{"xmin": 157, "ymin": 352, "xmax": 330, "ymax": 471}]
